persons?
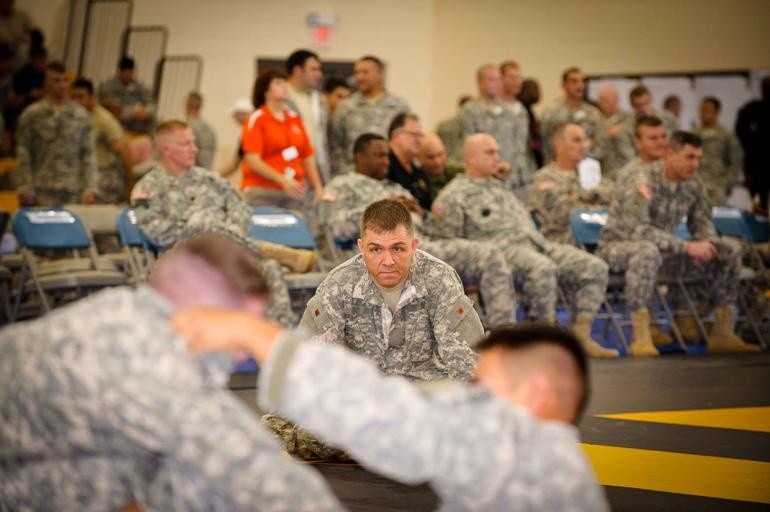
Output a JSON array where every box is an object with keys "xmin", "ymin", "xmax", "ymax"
[
  {"xmin": 2, "ymin": 236, "xmax": 349, "ymax": 512},
  {"xmin": 254, "ymin": 198, "xmax": 489, "ymax": 460},
  {"xmin": 2, "ymin": 47, "xmax": 769, "ymax": 363},
  {"xmin": 169, "ymin": 304, "xmax": 609, "ymax": 512}
]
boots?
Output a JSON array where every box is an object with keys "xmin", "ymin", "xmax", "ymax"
[
  {"xmin": 573, "ymin": 305, "xmax": 761, "ymax": 358},
  {"xmin": 260, "ymin": 243, "xmax": 316, "ymax": 273}
]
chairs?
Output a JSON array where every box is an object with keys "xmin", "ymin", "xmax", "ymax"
[{"xmin": 12, "ymin": 203, "xmax": 768, "ymax": 354}]
